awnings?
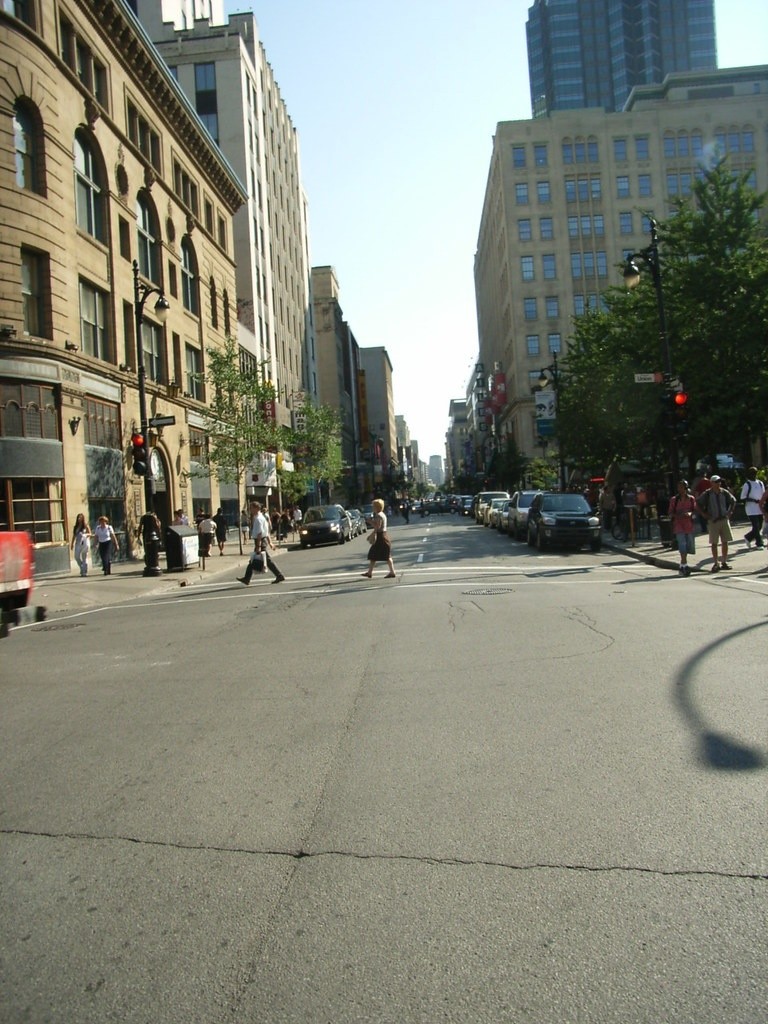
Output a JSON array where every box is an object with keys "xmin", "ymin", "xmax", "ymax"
[{"xmin": 590, "ymin": 477, "xmax": 606, "ymax": 483}]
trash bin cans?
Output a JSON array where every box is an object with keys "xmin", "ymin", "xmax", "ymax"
[{"xmin": 654, "ymin": 515, "xmax": 673, "ymax": 549}]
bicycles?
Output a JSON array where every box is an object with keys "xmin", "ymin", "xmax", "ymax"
[{"xmin": 611, "ymin": 507, "xmax": 630, "ymax": 542}]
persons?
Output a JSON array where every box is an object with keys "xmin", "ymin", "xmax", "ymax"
[
  {"xmin": 599, "ymin": 485, "xmax": 617, "ymax": 533},
  {"xmin": 695, "ymin": 474, "xmax": 737, "ymax": 573},
  {"xmin": 719, "ymin": 478, "xmax": 732, "ymax": 511},
  {"xmin": 360, "ymin": 498, "xmax": 396, "ymax": 578},
  {"xmin": 213, "ymin": 507, "xmax": 230, "ymax": 556},
  {"xmin": 138, "ymin": 508, "xmax": 189, "ymax": 554},
  {"xmin": 290, "ymin": 505, "xmax": 303, "ymax": 534},
  {"xmin": 740, "ymin": 465, "xmax": 768, "ymax": 551},
  {"xmin": 196, "ymin": 507, "xmax": 217, "ymax": 557},
  {"xmin": 421, "ymin": 499, "xmax": 426, "ymax": 517},
  {"xmin": 691, "ymin": 469, "xmax": 712, "ymax": 533},
  {"xmin": 235, "ymin": 501, "xmax": 284, "ymax": 584},
  {"xmin": 568, "ymin": 483, "xmax": 600, "ymax": 514},
  {"xmin": 668, "ymin": 479, "xmax": 698, "ymax": 576},
  {"xmin": 387, "ymin": 498, "xmax": 410, "ymax": 523},
  {"xmin": 613, "ymin": 479, "xmax": 648, "ymax": 530},
  {"xmin": 263, "ymin": 506, "xmax": 293, "ymax": 543},
  {"xmin": 93, "ymin": 516, "xmax": 120, "ymax": 575},
  {"xmin": 71, "ymin": 514, "xmax": 91, "ymax": 576}
]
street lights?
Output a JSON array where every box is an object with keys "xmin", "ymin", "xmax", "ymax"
[
  {"xmin": 537, "ymin": 349, "xmax": 566, "ymax": 493},
  {"xmin": 623, "ymin": 217, "xmax": 680, "ymax": 496},
  {"xmin": 133, "ymin": 259, "xmax": 170, "ymax": 578}
]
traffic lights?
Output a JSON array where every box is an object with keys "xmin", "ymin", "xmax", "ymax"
[
  {"xmin": 659, "ymin": 393, "xmax": 674, "ymax": 424},
  {"xmin": 131, "ymin": 433, "xmax": 146, "ymax": 475},
  {"xmin": 674, "ymin": 392, "xmax": 689, "ymax": 422}
]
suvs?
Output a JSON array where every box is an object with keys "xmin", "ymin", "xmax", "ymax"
[
  {"xmin": 527, "ymin": 491, "xmax": 602, "ymax": 553},
  {"xmin": 508, "ymin": 491, "xmax": 546, "ymax": 540},
  {"xmin": 297, "ymin": 504, "xmax": 352, "ymax": 548}
]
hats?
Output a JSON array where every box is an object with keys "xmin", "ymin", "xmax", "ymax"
[{"xmin": 710, "ymin": 474, "xmax": 721, "ymax": 482}]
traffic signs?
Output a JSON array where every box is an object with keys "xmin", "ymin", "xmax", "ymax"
[{"xmin": 149, "ymin": 416, "xmax": 175, "ymax": 427}]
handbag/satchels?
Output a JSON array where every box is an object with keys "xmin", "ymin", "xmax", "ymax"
[
  {"xmin": 251, "ymin": 551, "xmax": 268, "ymax": 574},
  {"xmin": 367, "ymin": 532, "xmax": 375, "ymax": 544}
]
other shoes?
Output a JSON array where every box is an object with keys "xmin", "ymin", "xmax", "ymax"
[
  {"xmin": 81, "ymin": 572, "xmax": 86, "ymax": 577},
  {"xmin": 721, "ymin": 563, "xmax": 733, "ymax": 569},
  {"xmin": 756, "ymin": 545, "xmax": 764, "ymax": 550},
  {"xmin": 236, "ymin": 577, "xmax": 249, "ymax": 585},
  {"xmin": 384, "ymin": 574, "xmax": 395, "ymax": 578},
  {"xmin": 272, "ymin": 575, "xmax": 285, "ymax": 584},
  {"xmin": 712, "ymin": 564, "xmax": 719, "ymax": 573},
  {"xmin": 361, "ymin": 573, "xmax": 372, "ymax": 578},
  {"xmin": 679, "ymin": 566, "xmax": 691, "ymax": 575},
  {"xmin": 744, "ymin": 536, "xmax": 751, "ymax": 548}
]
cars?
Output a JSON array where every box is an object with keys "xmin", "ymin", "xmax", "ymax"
[
  {"xmin": 346, "ymin": 504, "xmax": 373, "ymax": 540},
  {"xmin": 696, "ymin": 453, "xmax": 747, "ymax": 476},
  {"xmin": 447, "ymin": 491, "xmax": 514, "ymax": 534},
  {"xmin": 423, "ymin": 501, "xmax": 461, "ymax": 516},
  {"xmin": 411, "ymin": 492, "xmax": 445, "ymax": 514}
]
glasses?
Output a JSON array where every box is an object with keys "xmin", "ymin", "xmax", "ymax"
[{"xmin": 715, "ymin": 479, "xmax": 721, "ymax": 483}]
